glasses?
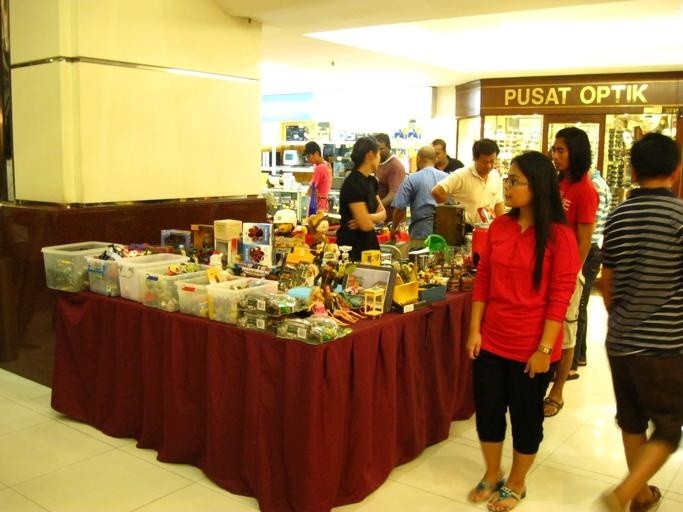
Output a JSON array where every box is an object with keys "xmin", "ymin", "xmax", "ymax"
[{"xmin": 503, "ymin": 178, "xmax": 529, "ymax": 186}]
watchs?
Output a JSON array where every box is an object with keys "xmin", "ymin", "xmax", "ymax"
[{"xmin": 535, "ymin": 342, "xmax": 555, "ymax": 356}]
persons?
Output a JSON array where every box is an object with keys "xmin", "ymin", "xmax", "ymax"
[
  {"xmin": 428, "ymin": 138, "xmax": 506, "ymax": 243},
  {"xmin": 384, "ymin": 145, "xmax": 453, "ymax": 242},
  {"xmin": 368, "ymin": 133, "xmax": 408, "ymax": 224},
  {"xmin": 545, "ymin": 127, "xmax": 599, "ymax": 418},
  {"xmin": 546, "ymin": 160, "xmax": 611, "ymax": 384},
  {"xmin": 301, "ymin": 140, "xmax": 334, "ymax": 226},
  {"xmin": 333, "ymin": 134, "xmax": 387, "ymax": 261},
  {"xmin": 460, "ymin": 152, "xmax": 580, "ymax": 509},
  {"xmin": 594, "ymin": 129, "xmax": 683, "ymax": 510},
  {"xmin": 414, "ymin": 138, "xmax": 463, "ymax": 172}
]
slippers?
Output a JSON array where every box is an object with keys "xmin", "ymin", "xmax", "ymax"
[
  {"xmin": 544, "ymin": 397, "xmax": 563, "ymax": 416},
  {"xmin": 551, "ymin": 369, "xmax": 579, "ymax": 381},
  {"xmin": 574, "ymin": 358, "xmax": 586, "ymax": 366},
  {"xmin": 468, "ymin": 479, "xmax": 505, "ymax": 505},
  {"xmin": 487, "ymin": 486, "xmax": 526, "ymax": 512}
]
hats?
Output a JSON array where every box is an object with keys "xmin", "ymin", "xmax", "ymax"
[{"xmin": 302, "ymin": 141, "xmax": 319, "ymax": 158}]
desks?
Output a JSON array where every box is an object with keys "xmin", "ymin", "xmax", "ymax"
[{"xmin": 50, "ymin": 283, "xmax": 474, "ymax": 496}]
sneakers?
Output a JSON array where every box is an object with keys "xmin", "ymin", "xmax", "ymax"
[{"xmin": 629, "ymin": 485, "xmax": 661, "ymax": 512}]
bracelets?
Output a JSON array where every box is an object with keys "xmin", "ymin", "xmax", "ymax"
[{"xmin": 389, "ymin": 227, "xmax": 397, "ymax": 233}]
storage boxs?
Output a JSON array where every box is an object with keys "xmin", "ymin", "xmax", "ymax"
[{"xmin": 41, "ymin": 234, "xmax": 278, "ymax": 331}]
